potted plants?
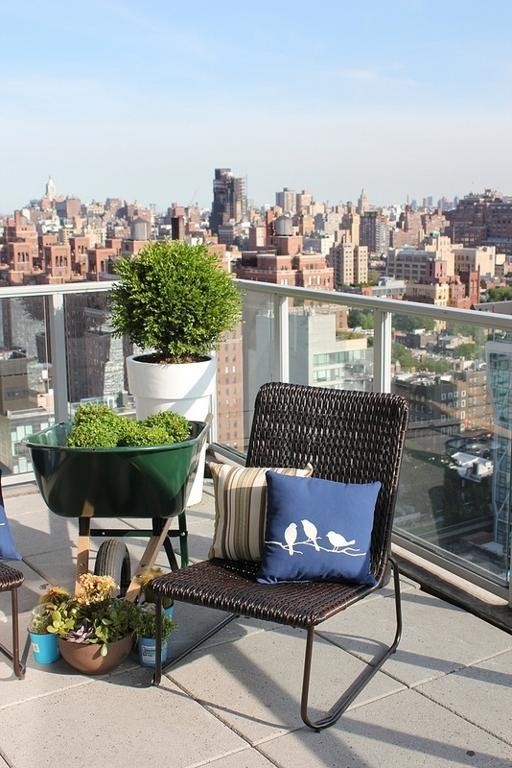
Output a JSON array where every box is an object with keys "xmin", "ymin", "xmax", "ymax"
[
  {"xmin": 136, "ymin": 608, "xmax": 178, "ymax": 667},
  {"xmin": 26, "ymin": 602, "xmax": 60, "ymax": 664},
  {"xmin": 103, "ymin": 234, "xmax": 248, "ymax": 505},
  {"xmin": 135, "ymin": 569, "xmax": 172, "ymax": 628},
  {"xmin": 21, "ymin": 399, "xmax": 214, "ymax": 519}
]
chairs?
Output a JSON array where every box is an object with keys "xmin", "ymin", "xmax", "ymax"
[
  {"xmin": 147, "ymin": 381, "xmax": 410, "ymax": 733},
  {"xmin": 1, "ymin": 470, "xmax": 25, "ymax": 681}
]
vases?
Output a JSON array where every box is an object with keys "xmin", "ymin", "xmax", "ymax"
[{"xmin": 57, "ymin": 631, "xmax": 137, "ymax": 673}]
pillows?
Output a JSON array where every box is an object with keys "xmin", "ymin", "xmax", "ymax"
[
  {"xmin": 1, "ymin": 505, "xmax": 22, "ymax": 561},
  {"xmin": 256, "ymin": 471, "xmax": 382, "ymax": 586},
  {"xmin": 205, "ymin": 461, "xmax": 313, "ymax": 561}
]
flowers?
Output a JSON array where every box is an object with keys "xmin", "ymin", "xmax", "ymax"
[{"xmin": 45, "ymin": 571, "xmax": 144, "ymax": 656}]
